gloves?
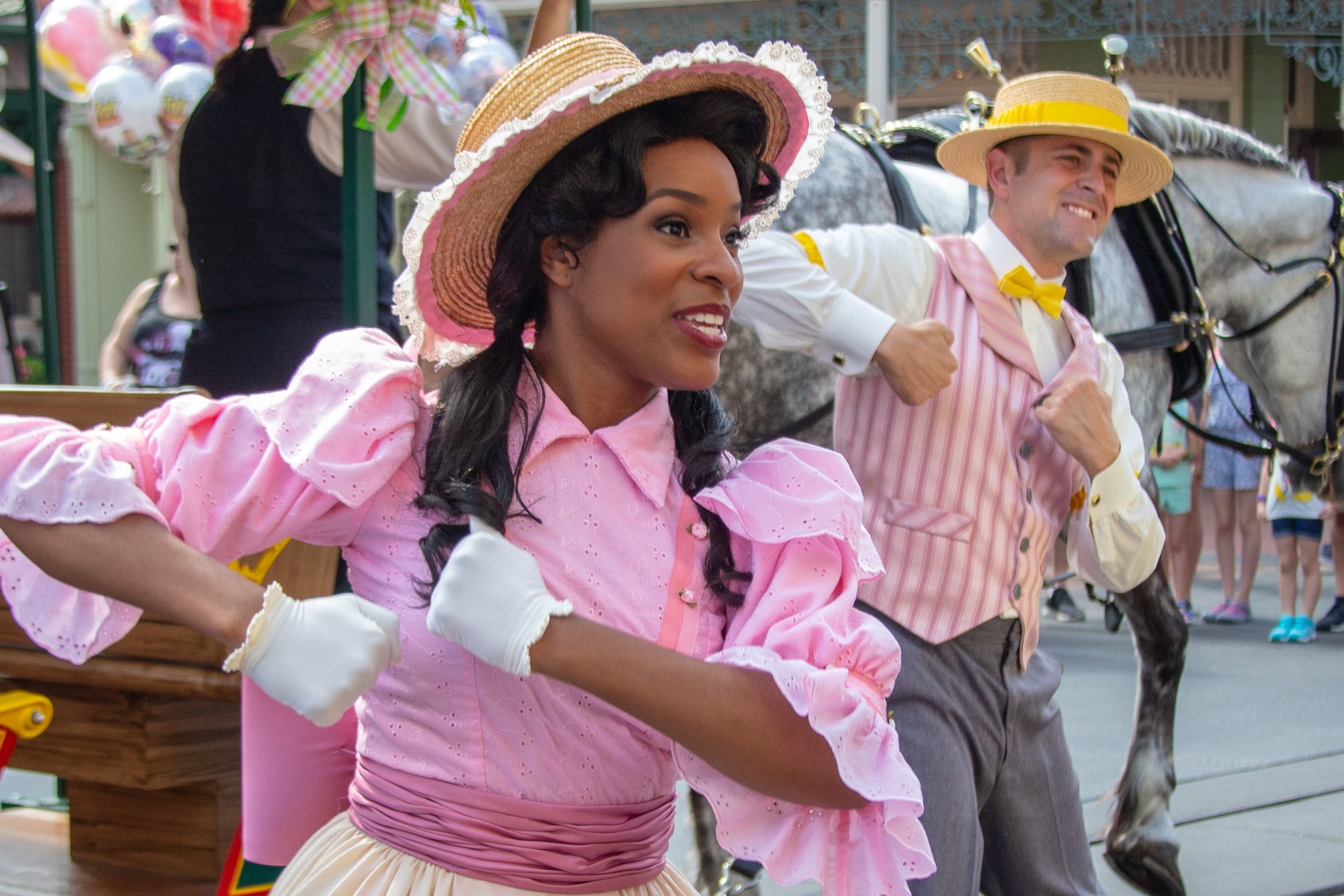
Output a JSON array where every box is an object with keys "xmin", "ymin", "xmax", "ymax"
[
  {"xmin": 427, "ymin": 530, "xmax": 574, "ymax": 680},
  {"xmin": 220, "ymin": 579, "xmax": 401, "ymax": 727}
]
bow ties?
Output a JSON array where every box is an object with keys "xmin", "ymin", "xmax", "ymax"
[{"xmin": 995, "ymin": 266, "xmax": 1068, "ymax": 320}]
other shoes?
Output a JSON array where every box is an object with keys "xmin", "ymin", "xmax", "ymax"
[
  {"xmin": 1267, "ymin": 612, "xmax": 1318, "ymax": 643},
  {"xmin": 1176, "ymin": 600, "xmax": 1194, "ymax": 627},
  {"xmin": 1043, "ymin": 588, "xmax": 1085, "ymax": 622},
  {"xmin": 1200, "ymin": 597, "xmax": 1252, "ymax": 624},
  {"xmin": 1314, "ymin": 600, "xmax": 1344, "ymax": 634}
]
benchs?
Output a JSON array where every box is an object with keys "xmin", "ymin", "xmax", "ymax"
[{"xmin": 0, "ymin": 389, "xmax": 340, "ymax": 880}]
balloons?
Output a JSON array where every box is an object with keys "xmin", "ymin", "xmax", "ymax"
[
  {"xmin": 403, "ymin": 0, "xmax": 522, "ymax": 111},
  {"xmin": 35, "ymin": 0, "xmax": 251, "ymax": 164}
]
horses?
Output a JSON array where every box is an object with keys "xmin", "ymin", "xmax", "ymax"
[{"xmin": 713, "ymin": 95, "xmax": 1343, "ymax": 896}]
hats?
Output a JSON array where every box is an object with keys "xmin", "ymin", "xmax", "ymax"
[
  {"xmin": 934, "ymin": 70, "xmax": 1173, "ymax": 210},
  {"xmin": 389, "ymin": 34, "xmax": 832, "ymax": 373}
]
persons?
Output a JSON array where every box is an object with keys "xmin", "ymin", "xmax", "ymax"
[
  {"xmin": 100, "ymin": 237, "xmax": 202, "ymax": 388},
  {"xmin": 0, "ymin": 32, "xmax": 939, "ymax": 896},
  {"xmin": 1041, "ymin": 349, "xmax": 1343, "ymax": 644},
  {"xmin": 179, "ymin": 0, "xmax": 577, "ymax": 397},
  {"xmin": 726, "ymin": 73, "xmax": 1173, "ymax": 896}
]
